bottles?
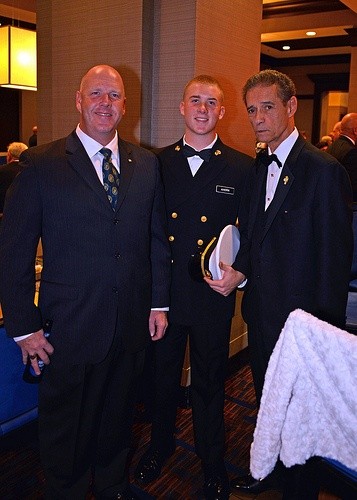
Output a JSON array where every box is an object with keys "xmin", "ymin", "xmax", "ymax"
[{"xmin": 22, "ymin": 320, "xmax": 53, "ymax": 384}]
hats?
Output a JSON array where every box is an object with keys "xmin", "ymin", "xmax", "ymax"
[{"xmin": 189, "ymin": 225, "xmax": 247, "ymax": 288}]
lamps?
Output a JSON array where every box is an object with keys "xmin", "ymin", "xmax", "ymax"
[{"xmin": 0, "ymin": 0, "xmax": 37, "ymax": 91}]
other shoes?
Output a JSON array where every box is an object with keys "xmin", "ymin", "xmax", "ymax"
[{"xmin": 112, "ymin": 487, "xmax": 139, "ymax": 500}]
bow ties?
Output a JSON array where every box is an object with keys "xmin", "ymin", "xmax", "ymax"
[
  {"xmin": 262, "ymin": 154, "xmax": 282, "ymax": 168},
  {"xmin": 182, "ymin": 145, "xmax": 212, "ymax": 164}
]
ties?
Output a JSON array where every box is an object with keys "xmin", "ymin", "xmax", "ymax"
[{"xmin": 99, "ymin": 148, "xmax": 120, "ymax": 213}]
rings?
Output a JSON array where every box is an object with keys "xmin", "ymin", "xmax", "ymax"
[{"xmin": 29, "ymin": 354, "xmax": 36, "ymax": 359}]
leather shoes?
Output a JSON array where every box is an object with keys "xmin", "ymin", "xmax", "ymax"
[
  {"xmin": 203, "ymin": 458, "xmax": 230, "ymax": 500},
  {"xmin": 230, "ymin": 475, "xmax": 276, "ymax": 493},
  {"xmin": 135, "ymin": 434, "xmax": 175, "ymax": 483}
]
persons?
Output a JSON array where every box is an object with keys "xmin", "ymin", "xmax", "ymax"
[
  {"xmin": 135, "ymin": 74, "xmax": 257, "ymax": 500},
  {"xmin": 241, "ymin": 70, "xmax": 357, "ymax": 500},
  {"xmin": 0, "ymin": 126, "xmax": 38, "ymax": 211},
  {"xmin": 0, "ymin": 64, "xmax": 172, "ymax": 500}
]
